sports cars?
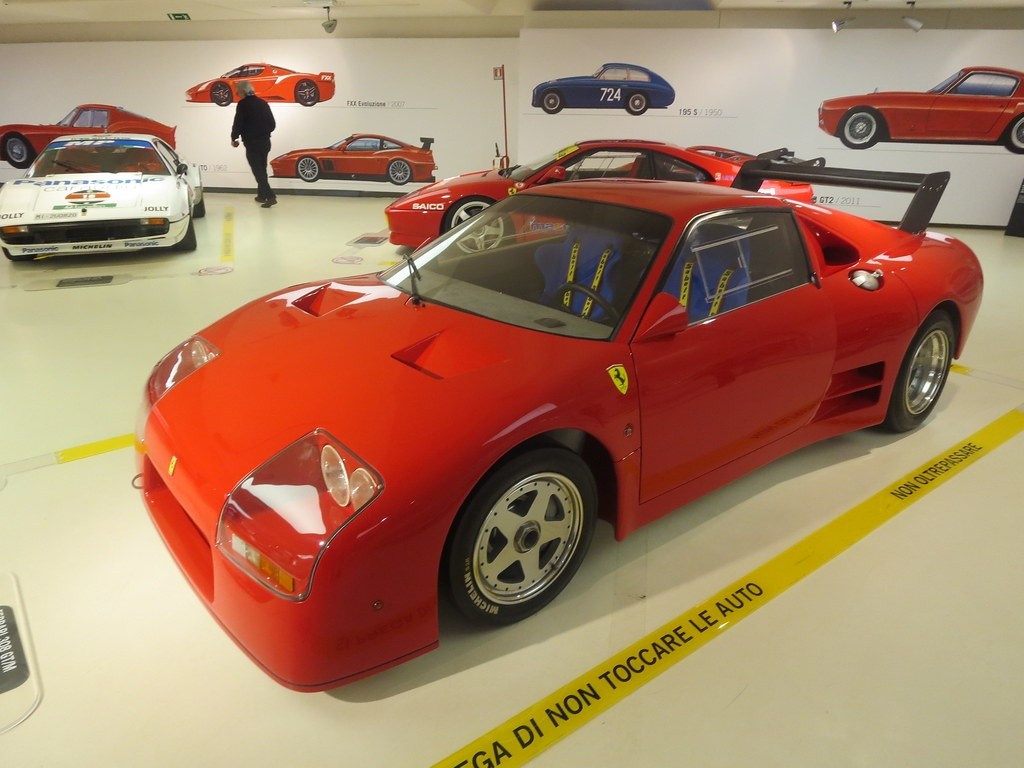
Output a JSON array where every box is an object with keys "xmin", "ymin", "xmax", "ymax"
[
  {"xmin": 130, "ymin": 179, "xmax": 985, "ymax": 693},
  {"xmin": 0, "ymin": 132, "xmax": 206, "ymax": 262},
  {"xmin": 384, "ymin": 139, "xmax": 952, "ymax": 259}
]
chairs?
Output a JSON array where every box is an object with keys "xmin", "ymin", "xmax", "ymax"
[
  {"xmin": 115, "ymin": 148, "xmax": 164, "ymax": 173},
  {"xmin": 533, "ymin": 227, "xmax": 626, "ymax": 321},
  {"xmin": 50, "ymin": 149, "xmax": 101, "ymax": 174},
  {"xmin": 662, "ymin": 224, "xmax": 752, "ymax": 323}
]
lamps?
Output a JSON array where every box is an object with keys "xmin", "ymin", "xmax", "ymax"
[
  {"xmin": 321, "ymin": 7, "xmax": 337, "ymax": 33},
  {"xmin": 901, "ymin": 5, "xmax": 924, "ymax": 33},
  {"xmin": 831, "ymin": 4, "xmax": 856, "ymax": 33}
]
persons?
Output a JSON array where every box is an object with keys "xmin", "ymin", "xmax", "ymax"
[{"xmin": 231, "ymin": 80, "xmax": 278, "ymax": 208}]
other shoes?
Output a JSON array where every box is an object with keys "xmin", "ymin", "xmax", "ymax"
[
  {"xmin": 255, "ymin": 196, "xmax": 268, "ymax": 203},
  {"xmin": 262, "ymin": 199, "xmax": 278, "ymax": 208}
]
